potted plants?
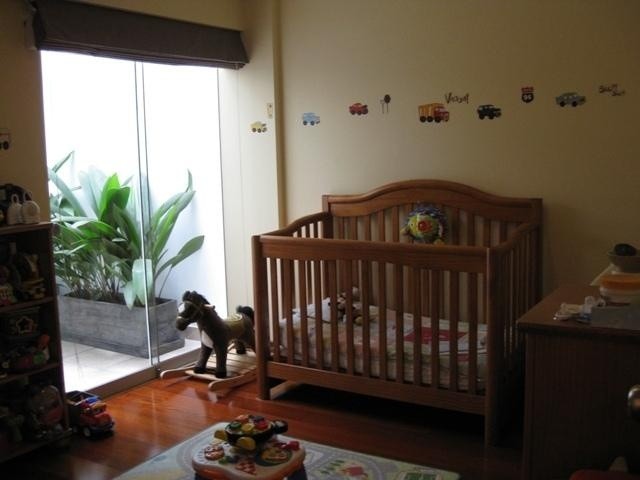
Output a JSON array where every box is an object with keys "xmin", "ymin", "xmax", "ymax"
[{"xmin": 43, "ymin": 173, "xmax": 205, "ymax": 360}]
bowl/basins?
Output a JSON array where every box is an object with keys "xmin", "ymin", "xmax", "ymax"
[{"xmin": 605, "ymin": 249, "xmax": 640, "ymax": 273}]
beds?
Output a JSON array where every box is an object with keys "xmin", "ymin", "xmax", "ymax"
[{"xmin": 250, "ymin": 177, "xmax": 544, "ymax": 446}]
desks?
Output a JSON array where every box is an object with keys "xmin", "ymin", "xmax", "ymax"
[{"xmin": 515, "ymin": 279, "xmax": 639, "ymax": 479}]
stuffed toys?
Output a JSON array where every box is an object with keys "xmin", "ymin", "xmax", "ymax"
[{"xmin": 328, "ymin": 300, "xmax": 376, "ymax": 325}]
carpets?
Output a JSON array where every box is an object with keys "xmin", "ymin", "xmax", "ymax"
[{"xmin": 105, "ymin": 422, "xmax": 461, "ymax": 480}]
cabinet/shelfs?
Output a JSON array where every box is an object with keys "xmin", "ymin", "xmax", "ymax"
[{"xmin": 0, "ymin": 220, "xmax": 76, "ymax": 464}]
